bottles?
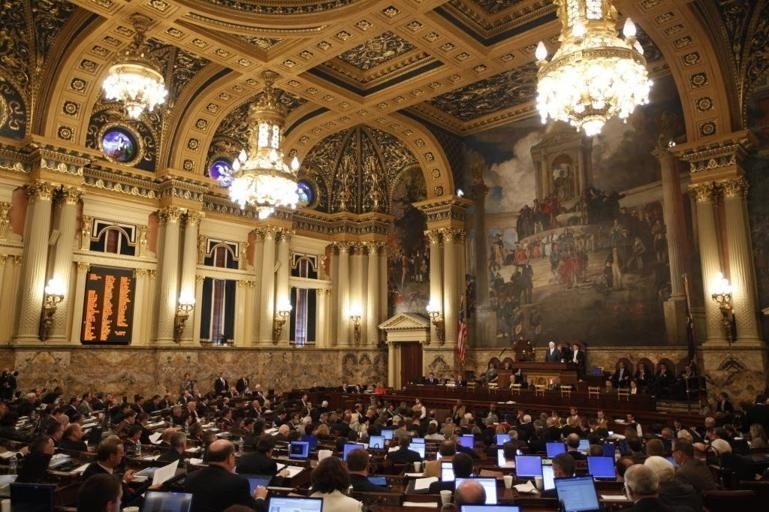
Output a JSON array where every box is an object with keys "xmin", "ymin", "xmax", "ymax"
[{"xmin": 136, "ymin": 440, "xmax": 142, "ymax": 457}]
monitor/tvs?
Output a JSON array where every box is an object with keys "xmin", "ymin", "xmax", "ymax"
[
  {"xmin": 302, "ymin": 436, "xmax": 316, "ymax": 450},
  {"xmin": 459, "ymin": 504, "xmax": 520, "ymax": 512},
  {"xmin": 289, "ymin": 440, "xmax": 309, "ymax": 458},
  {"xmin": 246, "ymin": 474, "xmax": 272, "ymax": 495},
  {"xmin": 266, "ymin": 496, "xmax": 324, "ymax": 512},
  {"xmin": 455, "ymin": 476, "xmax": 498, "ymax": 505},
  {"xmin": 139, "ymin": 490, "xmax": 193, "ymax": 512},
  {"xmin": 554, "ymin": 475, "xmax": 603, "ymax": 512},
  {"xmin": 665, "ymin": 455, "xmax": 680, "ymax": 468},
  {"xmin": 343, "ymin": 429, "xmax": 617, "ymax": 486}
]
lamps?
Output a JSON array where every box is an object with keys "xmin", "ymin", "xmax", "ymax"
[
  {"xmin": 346, "ymin": 300, "xmax": 365, "ymax": 348},
  {"xmin": 528, "ymin": 1, "xmax": 655, "ymax": 140},
  {"xmin": 100, "ymin": 11, "xmax": 171, "ymax": 120},
  {"xmin": 707, "ymin": 271, "xmax": 739, "ymax": 326},
  {"xmin": 225, "ymin": 68, "xmax": 303, "ymax": 223},
  {"xmin": 171, "ymin": 280, "xmax": 197, "ymax": 343},
  {"xmin": 36, "ymin": 274, "xmax": 67, "ymax": 342},
  {"xmin": 423, "ymin": 296, "xmax": 447, "ymax": 346},
  {"xmin": 269, "ymin": 291, "xmax": 294, "ymax": 345}
]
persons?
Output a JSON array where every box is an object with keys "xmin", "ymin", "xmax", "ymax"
[{"xmin": 0, "ymin": 339, "xmax": 768, "ymax": 511}]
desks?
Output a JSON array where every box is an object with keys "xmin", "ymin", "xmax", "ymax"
[{"xmin": 0, "ymin": 336, "xmax": 741, "ymax": 510}]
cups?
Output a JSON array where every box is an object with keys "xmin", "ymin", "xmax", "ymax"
[
  {"xmin": 534, "ymin": 477, "xmax": 543, "ymax": 490},
  {"xmin": 439, "ymin": 490, "xmax": 453, "ymax": 506},
  {"xmin": 414, "ymin": 462, "xmax": 421, "ymax": 472},
  {"xmin": 9, "ymin": 455, "xmax": 17, "ymax": 479},
  {"xmin": 422, "ymin": 460, "xmax": 428, "ymax": 472},
  {"xmin": 503, "ymin": 474, "xmax": 513, "ymax": 489}
]
